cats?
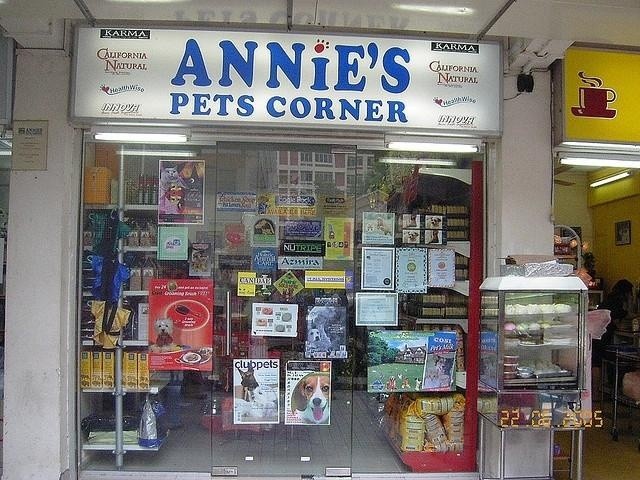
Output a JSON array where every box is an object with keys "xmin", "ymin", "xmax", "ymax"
[{"xmin": 161, "ymin": 165, "xmax": 186, "ymax": 188}]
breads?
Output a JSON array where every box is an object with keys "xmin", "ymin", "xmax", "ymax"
[{"xmin": 504, "ymin": 303, "xmax": 571, "ymax": 330}]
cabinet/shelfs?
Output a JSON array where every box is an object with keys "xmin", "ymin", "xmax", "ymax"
[
  {"xmin": 80, "ymin": 142, "xmax": 193, "ymax": 472},
  {"xmin": 476, "ymin": 272, "xmax": 591, "ymax": 480},
  {"xmin": 355, "ymin": 160, "xmax": 485, "ymax": 472}
]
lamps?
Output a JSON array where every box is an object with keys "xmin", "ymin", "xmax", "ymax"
[
  {"xmin": 90, "ymin": 131, "xmax": 190, "ymax": 143},
  {"xmin": 385, "ymin": 141, "xmax": 481, "ymax": 153},
  {"xmin": 588, "ymin": 168, "xmax": 633, "ymax": 188},
  {"xmin": 114, "ymin": 148, "xmax": 201, "ymax": 158}
]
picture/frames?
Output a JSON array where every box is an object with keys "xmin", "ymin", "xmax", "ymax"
[{"xmin": 614, "ymin": 219, "xmax": 632, "ymax": 246}]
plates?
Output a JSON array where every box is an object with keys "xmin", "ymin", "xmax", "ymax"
[{"xmin": 183, "ymin": 352, "xmax": 202, "ymax": 363}]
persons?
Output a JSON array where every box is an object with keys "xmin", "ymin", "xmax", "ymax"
[{"xmin": 592, "ymin": 279, "xmax": 634, "ymax": 400}]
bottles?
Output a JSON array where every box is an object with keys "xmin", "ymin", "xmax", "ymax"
[{"xmin": 137, "ymin": 170, "xmax": 157, "ymax": 205}]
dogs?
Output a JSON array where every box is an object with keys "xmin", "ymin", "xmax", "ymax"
[
  {"xmin": 237, "ymin": 360, "xmax": 259, "ymax": 390},
  {"xmin": 148, "ymin": 316, "xmax": 176, "ymax": 353},
  {"xmin": 307, "ymin": 323, "xmax": 323, "ymax": 344},
  {"xmin": 432, "ymin": 354, "xmax": 454, "ymax": 388},
  {"xmin": 408, "ymin": 231, "xmax": 419, "ymax": 244},
  {"xmin": 430, "ymin": 216, "xmax": 441, "ymax": 230},
  {"xmin": 407, "ymin": 214, "xmax": 417, "ymax": 227},
  {"xmin": 291, "ymin": 375, "xmax": 330, "ymax": 425}
]
